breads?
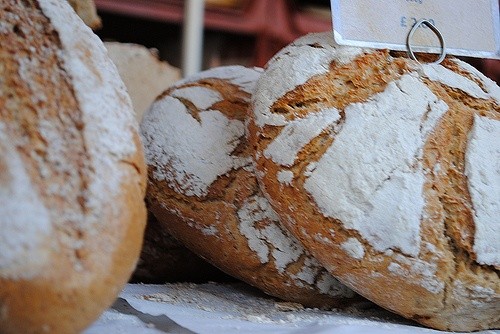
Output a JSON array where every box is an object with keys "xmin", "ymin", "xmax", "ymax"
[
  {"xmin": 0, "ymin": 0, "xmax": 149, "ymax": 334},
  {"xmin": 245, "ymin": 31, "xmax": 500, "ymax": 334},
  {"xmin": 139, "ymin": 65, "xmax": 357, "ymax": 308}
]
